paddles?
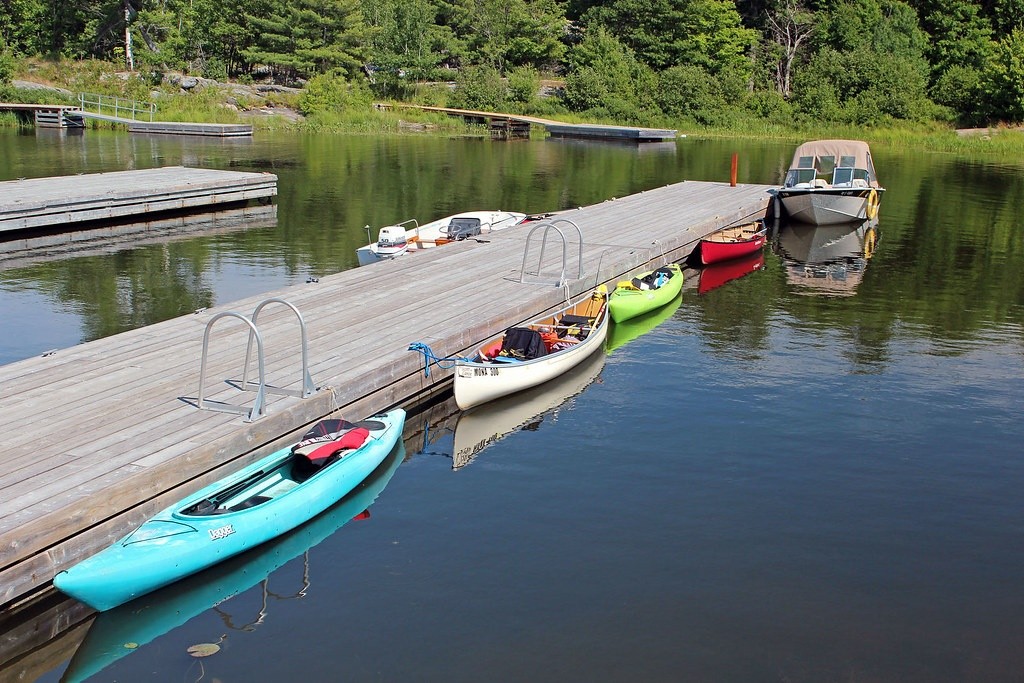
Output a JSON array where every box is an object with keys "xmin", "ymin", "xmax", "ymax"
[
  {"xmin": 586, "ymin": 301, "xmax": 607, "ymax": 337},
  {"xmin": 189, "ymin": 456, "xmax": 295, "ymax": 516},
  {"xmin": 739, "ymin": 227, "xmax": 771, "ymax": 241}
]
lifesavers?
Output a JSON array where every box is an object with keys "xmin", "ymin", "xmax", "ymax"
[
  {"xmin": 863, "ymin": 228, "xmax": 876, "ymax": 256},
  {"xmin": 866, "ymin": 190, "xmax": 879, "ymax": 221}
]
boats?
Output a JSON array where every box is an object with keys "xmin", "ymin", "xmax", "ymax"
[
  {"xmin": 56, "ymin": 434, "xmax": 406, "ymax": 683},
  {"xmin": 777, "ymin": 139, "xmax": 886, "ymax": 225},
  {"xmin": 451, "ymin": 283, "xmax": 609, "ymax": 413},
  {"xmin": 608, "ymin": 264, "xmax": 683, "ymax": 324},
  {"xmin": 605, "ymin": 290, "xmax": 684, "ymax": 350},
  {"xmin": 772, "ymin": 215, "xmax": 880, "ymax": 299},
  {"xmin": 451, "ymin": 337, "xmax": 606, "ymax": 471},
  {"xmin": 696, "ymin": 248, "xmax": 765, "ymax": 295},
  {"xmin": 51, "ymin": 406, "xmax": 406, "ymax": 613},
  {"xmin": 355, "ymin": 209, "xmax": 528, "ymax": 268},
  {"xmin": 698, "ymin": 217, "xmax": 767, "ymax": 265}
]
